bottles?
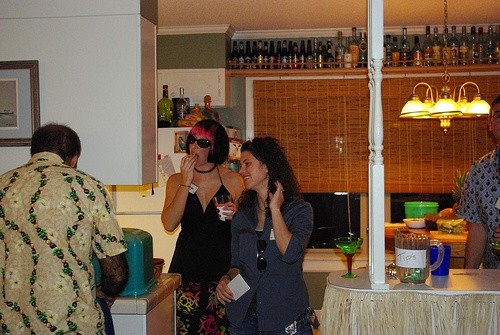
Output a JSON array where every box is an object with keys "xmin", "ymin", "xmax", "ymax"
[
  {"xmin": 229, "ymin": 25, "xmax": 368, "ymax": 67},
  {"xmin": 157, "ymin": 83, "xmax": 221, "ymax": 127},
  {"xmin": 381, "ymin": 24, "xmax": 500, "ymax": 68}
]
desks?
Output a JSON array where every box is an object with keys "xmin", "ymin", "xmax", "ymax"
[{"xmin": 321, "ymin": 269, "xmax": 500, "ymax": 335}]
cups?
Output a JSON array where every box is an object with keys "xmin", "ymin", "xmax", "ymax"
[
  {"xmin": 395, "ymin": 230, "xmax": 443, "ymax": 283},
  {"xmin": 214, "ymin": 194, "xmax": 233, "ymax": 220},
  {"xmin": 430, "ymin": 244, "xmax": 450, "ymax": 277}
]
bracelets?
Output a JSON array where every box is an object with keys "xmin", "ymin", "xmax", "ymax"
[{"xmin": 179, "ymin": 184, "xmax": 191, "ymax": 188}]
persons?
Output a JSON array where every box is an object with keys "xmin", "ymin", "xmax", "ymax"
[
  {"xmin": 0, "ymin": 124, "xmax": 129, "ymax": 335},
  {"xmin": 458, "ymin": 95, "xmax": 500, "ymax": 269},
  {"xmin": 162, "ymin": 119, "xmax": 246, "ymax": 335},
  {"xmin": 216, "ymin": 137, "xmax": 319, "ymax": 335}
]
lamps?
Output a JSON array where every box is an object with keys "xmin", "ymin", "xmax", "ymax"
[{"xmin": 400, "ymin": 0, "xmax": 490, "ymax": 134}]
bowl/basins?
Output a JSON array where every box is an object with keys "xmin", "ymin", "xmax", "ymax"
[
  {"xmin": 423, "ymin": 213, "xmax": 445, "ymax": 231},
  {"xmin": 437, "ymin": 216, "xmax": 465, "ymax": 233},
  {"xmin": 404, "ymin": 218, "xmax": 425, "ymax": 228},
  {"xmin": 405, "ymin": 202, "xmax": 439, "ymax": 218},
  {"xmin": 153, "ymin": 257, "xmax": 165, "ymax": 279}
]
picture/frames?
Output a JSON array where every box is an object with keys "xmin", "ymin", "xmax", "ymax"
[{"xmin": 0, "ymin": 59, "xmax": 41, "ymax": 148}]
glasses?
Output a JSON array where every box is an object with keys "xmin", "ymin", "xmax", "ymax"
[
  {"xmin": 491, "ymin": 111, "xmax": 500, "ymax": 121},
  {"xmin": 188, "ymin": 135, "xmax": 212, "ymax": 148},
  {"xmin": 256, "ymin": 240, "xmax": 266, "ymax": 271}
]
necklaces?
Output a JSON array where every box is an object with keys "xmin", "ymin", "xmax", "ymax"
[{"xmin": 194, "ymin": 163, "xmax": 216, "ymax": 173}]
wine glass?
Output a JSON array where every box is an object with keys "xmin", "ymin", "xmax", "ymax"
[{"xmin": 335, "ymin": 236, "xmax": 363, "ymax": 280}]
